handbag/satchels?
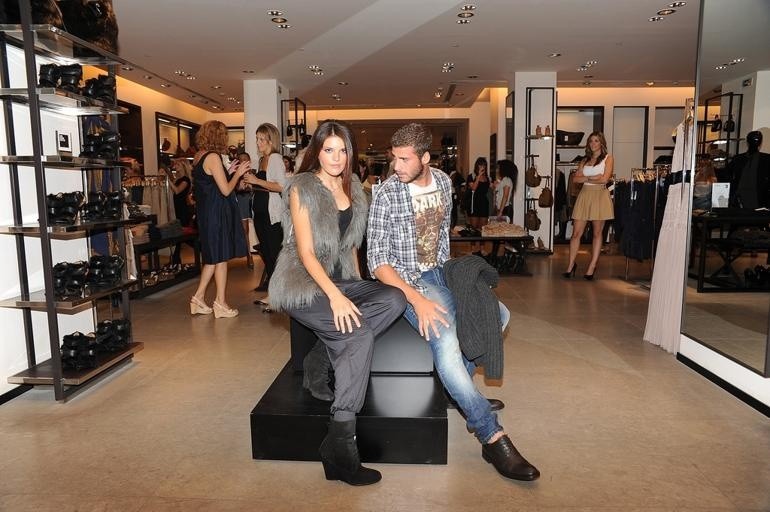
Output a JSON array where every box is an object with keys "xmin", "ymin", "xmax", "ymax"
[
  {"xmin": 87, "ymin": 254, "xmax": 125, "ymax": 289},
  {"xmin": 81, "ymin": 132, "xmax": 120, "ymax": 161},
  {"xmin": 524, "ymin": 209, "xmax": 541, "ymax": 232},
  {"xmin": 39, "ymin": 62, "xmax": 83, "ymax": 92},
  {"xmin": 82, "ymin": 75, "xmax": 117, "ymax": 105},
  {"xmin": 525, "ymin": 167, "xmax": 541, "ymax": 187},
  {"xmin": 538, "ymin": 188, "xmax": 554, "ymax": 207},
  {"xmin": 96, "ymin": 318, "xmax": 132, "ymax": 354},
  {"xmin": 45, "ymin": 192, "xmax": 82, "ymax": 226},
  {"xmin": 287, "ymin": 125, "xmax": 293, "ymax": 136},
  {"xmin": 299, "ymin": 123, "xmax": 305, "ymax": 136},
  {"xmin": 62, "ymin": 331, "xmax": 99, "ymax": 371},
  {"xmin": 50, "ymin": 259, "xmax": 89, "ymax": 299},
  {"xmin": 84, "ymin": 190, "xmax": 124, "ymax": 222}
]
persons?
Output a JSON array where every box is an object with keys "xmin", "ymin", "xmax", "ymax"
[
  {"xmin": 366, "ymin": 122, "xmax": 540, "ymax": 481},
  {"xmin": 723, "ymin": 130, "xmax": 770, "ymax": 211},
  {"xmin": 238, "ymin": 122, "xmax": 287, "ymax": 314},
  {"xmin": 157, "ymin": 147, "xmax": 518, "ymax": 269},
  {"xmin": 561, "ymin": 130, "xmax": 616, "ymax": 280},
  {"xmin": 189, "ymin": 120, "xmax": 251, "ymax": 318},
  {"xmin": 267, "ymin": 121, "xmax": 408, "ymax": 487}
]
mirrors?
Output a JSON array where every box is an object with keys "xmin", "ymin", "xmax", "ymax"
[
  {"xmin": 505, "ymin": 90, "xmax": 514, "ymax": 160},
  {"xmin": 680, "ymin": 1, "xmax": 770, "ymax": 377}
]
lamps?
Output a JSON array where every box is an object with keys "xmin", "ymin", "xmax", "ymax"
[
  {"xmin": 456, "ymin": 5, "xmax": 475, "ymax": 25},
  {"xmin": 268, "ymin": 9, "xmax": 294, "ymax": 29}
]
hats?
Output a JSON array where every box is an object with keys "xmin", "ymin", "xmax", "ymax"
[{"xmin": 746, "ymin": 130, "xmax": 762, "ymax": 146}]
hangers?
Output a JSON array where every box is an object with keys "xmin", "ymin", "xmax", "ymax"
[
  {"xmin": 630, "ymin": 167, "xmax": 657, "ymax": 182},
  {"xmin": 122, "ymin": 174, "xmax": 167, "ymax": 188},
  {"xmin": 672, "ymin": 98, "xmax": 695, "ymax": 137}
]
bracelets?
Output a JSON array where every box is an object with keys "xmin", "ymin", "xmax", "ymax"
[{"xmin": 587, "ymin": 176, "xmax": 592, "ymax": 183}]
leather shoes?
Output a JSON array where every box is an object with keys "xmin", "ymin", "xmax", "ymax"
[
  {"xmin": 254, "ymin": 300, "xmax": 269, "ymax": 306},
  {"xmin": 481, "ymin": 434, "xmax": 541, "ymax": 482},
  {"xmin": 447, "ymin": 397, "xmax": 505, "ymax": 412},
  {"xmin": 255, "ymin": 285, "xmax": 267, "ymax": 292},
  {"xmin": 262, "ymin": 307, "xmax": 272, "ymax": 313}
]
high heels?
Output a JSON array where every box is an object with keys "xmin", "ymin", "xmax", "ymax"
[
  {"xmin": 561, "ymin": 262, "xmax": 577, "ymax": 280},
  {"xmin": 528, "ymin": 243, "xmax": 535, "ymax": 249},
  {"xmin": 189, "ymin": 296, "xmax": 214, "ymax": 315},
  {"xmin": 246, "ymin": 257, "xmax": 256, "ymax": 270},
  {"xmin": 537, "ymin": 237, "xmax": 545, "ymax": 250},
  {"xmin": 213, "ymin": 300, "xmax": 239, "ymax": 319},
  {"xmin": 743, "ymin": 268, "xmax": 754, "ymax": 289},
  {"xmin": 756, "ymin": 266, "xmax": 766, "ymax": 280},
  {"xmin": 583, "ymin": 267, "xmax": 596, "ymax": 281}
]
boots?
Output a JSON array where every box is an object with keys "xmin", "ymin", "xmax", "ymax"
[
  {"xmin": 319, "ymin": 419, "xmax": 383, "ymax": 487},
  {"xmin": 302, "ymin": 339, "xmax": 335, "ymax": 403}
]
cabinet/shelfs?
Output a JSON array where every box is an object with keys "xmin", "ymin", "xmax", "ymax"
[
  {"xmin": 450, "ymin": 236, "xmax": 533, "ymax": 278},
  {"xmin": 0, "ymin": 24, "xmax": 146, "ymax": 403},
  {"xmin": 524, "ymin": 88, "xmax": 555, "ymax": 255},
  {"xmin": 127, "ymin": 232, "xmax": 199, "ymax": 299},
  {"xmin": 702, "ymin": 91, "xmax": 742, "ymax": 166},
  {"xmin": 554, "ymin": 105, "xmax": 604, "ymax": 245},
  {"xmin": 690, "ymin": 217, "xmax": 770, "ymax": 293},
  {"xmin": 280, "ymin": 97, "xmax": 307, "ymax": 165},
  {"xmin": 249, "ymin": 295, "xmax": 449, "ymax": 466},
  {"xmin": 127, "ymin": 213, "xmax": 160, "ymax": 275}
]
